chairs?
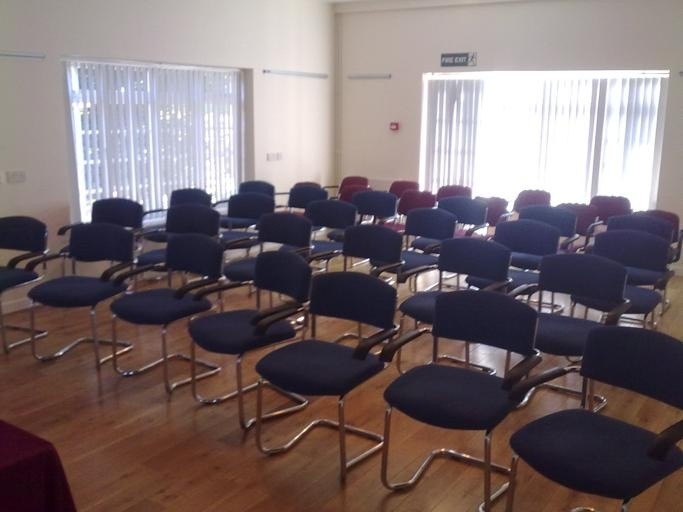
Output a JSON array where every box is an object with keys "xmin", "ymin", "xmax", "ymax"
[
  {"xmin": 0, "ymin": 215, "xmax": 52, "ymax": 352},
  {"xmin": 506, "ymin": 325, "xmax": 683, "ymax": 511},
  {"xmin": 380, "ymin": 290, "xmax": 542, "ymax": 510},
  {"xmin": 513, "ymin": 252, "xmax": 631, "ymax": 414},
  {"xmin": 395, "ymin": 237, "xmax": 514, "ymax": 379},
  {"xmin": 254, "ymin": 271, "xmax": 401, "ymax": 482},
  {"xmin": 58, "ymin": 176, "xmax": 682, "ymax": 355},
  {"xmin": 108, "ymin": 234, "xmax": 225, "ymax": 395},
  {"xmin": 27, "ymin": 222, "xmax": 139, "ymax": 366},
  {"xmin": 187, "ymin": 250, "xmax": 313, "ymax": 433}
]
